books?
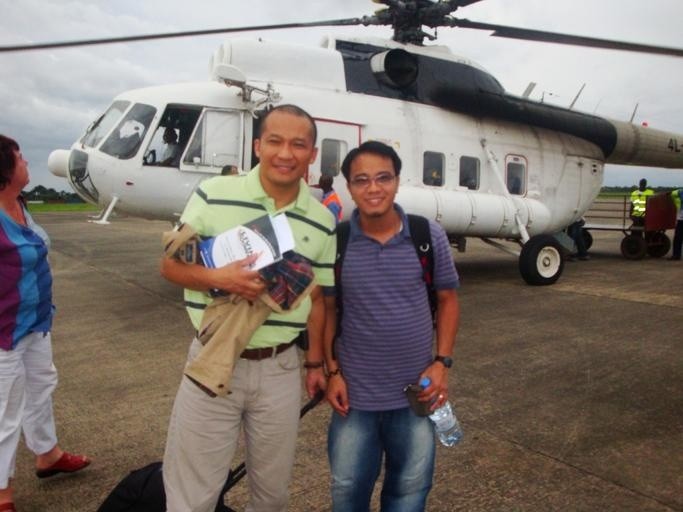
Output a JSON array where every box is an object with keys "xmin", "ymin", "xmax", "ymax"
[
  {"xmin": 198, "ymin": 213, "xmax": 284, "ymax": 292},
  {"xmin": 273, "ymin": 214, "xmax": 296, "ymax": 252}
]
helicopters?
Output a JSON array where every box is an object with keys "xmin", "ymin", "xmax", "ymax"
[{"xmin": 0, "ymin": 1, "xmax": 683, "ymax": 286}]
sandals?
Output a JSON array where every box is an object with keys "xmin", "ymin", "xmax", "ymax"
[
  {"xmin": 0, "ymin": 500, "xmax": 17, "ymax": 512},
  {"xmin": 36, "ymin": 451, "xmax": 92, "ymax": 478}
]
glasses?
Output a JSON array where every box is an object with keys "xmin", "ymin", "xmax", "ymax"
[{"xmin": 349, "ymin": 173, "xmax": 397, "ymax": 185}]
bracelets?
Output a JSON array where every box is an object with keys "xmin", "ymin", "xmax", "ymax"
[
  {"xmin": 325, "ymin": 367, "xmax": 340, "ymax": 378},
  {"xmin": 302, "ymin": 360, "xmax": 325, "ymax": 369}
]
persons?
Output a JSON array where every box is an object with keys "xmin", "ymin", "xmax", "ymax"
[
  {"xmin": 665, "ymin": 188, "xmax": 683, "ymax": 260},
  {"xmin": 160, "ymin": 100, "xmax": 338, "ymax": 510},
  {"xmin": 322, "ymin": 139, "xmax": 459, "ymax": 512},
  {"xmin": 221, "ymin": 165, "xmax": 237, "ymax": 175},
  {"xmin": 0, "ymin": 132, "xmax": 94, "ymax": 512},
  {"xmin": 319, "ymin": 173, "xmax": 343, "ymax": 227},
  {"xmin": 629, "ymin": 178, "xmax": 654, "ymax": 237}
]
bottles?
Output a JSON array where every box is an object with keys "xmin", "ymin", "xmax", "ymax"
[{"xmin": 419, "ymin": 377, "xmax": 463, "ymax": 448}]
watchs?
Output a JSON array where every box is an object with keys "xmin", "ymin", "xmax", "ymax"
[{"xmin": 435, "ymin": 355, "xmax": 452, "ymax": 368}]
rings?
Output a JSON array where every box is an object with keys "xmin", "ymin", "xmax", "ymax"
[{"xmin": 439, "ymin": 394, "xmax": 444, "ymax": 399}]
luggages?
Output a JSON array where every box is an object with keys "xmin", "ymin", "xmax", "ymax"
[{"xmin": 96, "ymin": 458, "xmax": 241, "ymax": 512}]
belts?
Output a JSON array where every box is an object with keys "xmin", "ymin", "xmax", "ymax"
[{"xmin": 194, "ymin": 329, "xmax": 302, "ymax": 361}]
